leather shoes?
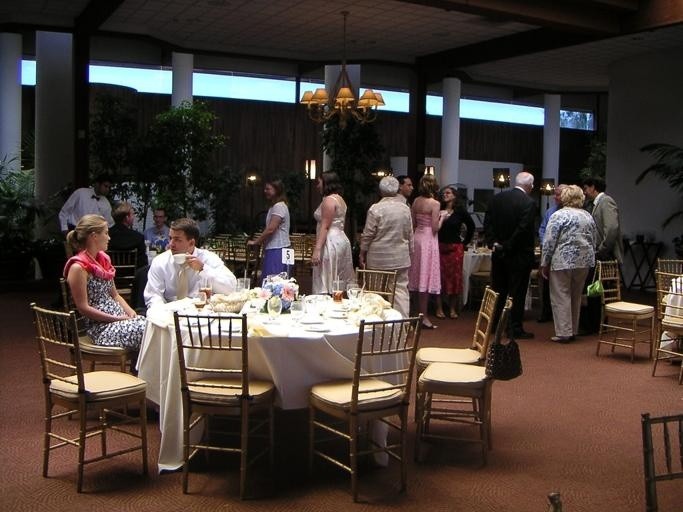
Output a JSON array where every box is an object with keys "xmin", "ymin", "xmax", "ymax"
[
  {"xmin": 550, "ymin": 334, "xmax": 575, "ymax": 342},
  {"xmin": 508, "ymin": 331, "xmax": 533, "ymax": 339}
]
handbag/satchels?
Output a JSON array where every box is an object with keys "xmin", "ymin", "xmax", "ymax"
[
  {"xmin": 234, "ymin": 263, "xmax": 255, "ymax": 278},
  {"xmin": 485, "ymin": 342, "xmax": 522, "ymax": 380},
  {"xmin": 587, "ymin": 280, "xmax": 604, "ymax": 298}
]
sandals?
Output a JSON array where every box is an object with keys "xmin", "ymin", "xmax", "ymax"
[{"xmin": 423, "ymin": 313, "xmax": 458, "ymax": 329}]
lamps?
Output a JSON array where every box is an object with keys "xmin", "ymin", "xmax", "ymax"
[
  {"xmin": 300, "ymin": 11, "xmax": 384, "ymax": 124},
  {"xmin": 492, "ymin": 168, "xmax": 511, "ymax": 193},
  {"xmin": 540, "ymin": 178, "xmax": 555, "ymax": 210}
]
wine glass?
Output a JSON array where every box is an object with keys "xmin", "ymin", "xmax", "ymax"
[{"xmin": 192, "ymin": 278, "xmax": 213, "ymax": 314}]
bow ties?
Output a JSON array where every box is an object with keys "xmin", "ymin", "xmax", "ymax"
[{"xmin": 91, "ymin": 195, "xmax": 99, "ymax": 200}]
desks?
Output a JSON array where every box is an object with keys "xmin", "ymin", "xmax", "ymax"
[{"xmin": 624, "ymin": 238, "xmax": 665, "ymax": 295}]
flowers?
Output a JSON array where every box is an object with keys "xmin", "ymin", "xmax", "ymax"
[{"xmin": 257, "ymin": 275, "xmax": 305, "ymax": 308}]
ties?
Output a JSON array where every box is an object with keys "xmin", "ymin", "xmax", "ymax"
[{"xmin": 176, "ymin": 263, "xmax": 187, "ymax": 300}]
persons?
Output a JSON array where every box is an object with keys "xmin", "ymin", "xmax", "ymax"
[
  {"xmin": 64, "ymin": 214, "xmax": 157, "ymax": 419},
  {"xmin": 246, "ymin": 178, "xmax": 291, "ymax": 286},
  {"xmin": 311, "ymin": 171, "xmax": 355, "ymax": 294},
  {"xmin": 108, "ymin": 203, "xmax": 148, "ymax": 289},
  {"xmin": 358, "ymin": 176, "xmax": 415, "ymax": 325},
  {"xmin": 144, "ymin": 209, "xmax": 170, "ymax": 249},
  {"xmin": 142, "ymin": 218, "xmax": 237, "ymax": 308},
  {"xmin": 53, "ymin": 176, "xmax": 116, "ymax": 307},
  {"xmin": 398, "ymin": 172, "xmax": 623, "ymax": 343}
]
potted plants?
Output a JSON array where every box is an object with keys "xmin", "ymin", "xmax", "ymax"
[{"xmin": 0, "ymin": 155, "xmax": 54, "ymax": 280}]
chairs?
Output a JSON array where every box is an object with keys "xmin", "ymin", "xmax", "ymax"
[
  {"xmin": 650, "ymin": 256, "xmax": 683, "ymax": 385},
  {"xmin": 173, "ymin": 309, "xmax": 279, "ymax": 506},
  {"xmin": 200, "ymin": 232, "xmax": 317, "ymax": 287},
  {"xmin": 60, "ymin": 276, "xmax": 138, "ymax": 425},
  {"xmin": 30, "ymin": 301, "xmax": 150, "ymax": 494},
  {"xmin": 638, "ymin": 409, "xmax": 681, "ymax": 511},
  {"xmin": 352, "ymin": 266, "xmax": 399, "ymax": 308},
  {"xmin": 105, "ymin": 245, "xmax": 138, "ymax": 305},
  {"xmin": 306, "ymin": 312, "xmax": 427, "ymax": 503},
  {"xmin": 415, "ymin": 284, "xmax": 500, "ymax": 390},
  {"xmin": 594, "ymin": 259, "xmax": 656, "ymax": 363},
  {"xmin": 459, "ymin": 247, "xmax": 553, "ymax": 324},
  {"xmin": 414, "ymin": 295, "xmax": 512, "ymax": 463}
]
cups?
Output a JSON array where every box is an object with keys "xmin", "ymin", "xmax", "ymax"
[
  {"xmin": 267, "ymin": 299, "xmax": 304, "ymax": 321},
  {"xmin": 173, "ymin": 253, "xmax": 187, "ymax": 266},
  {"xmin": 238, "ymin": 278, "xmax": 250, "ymax": 292},
  {"xmin": 331, "ymin": 280, "xmax": 362, "ymax": 305}
]
